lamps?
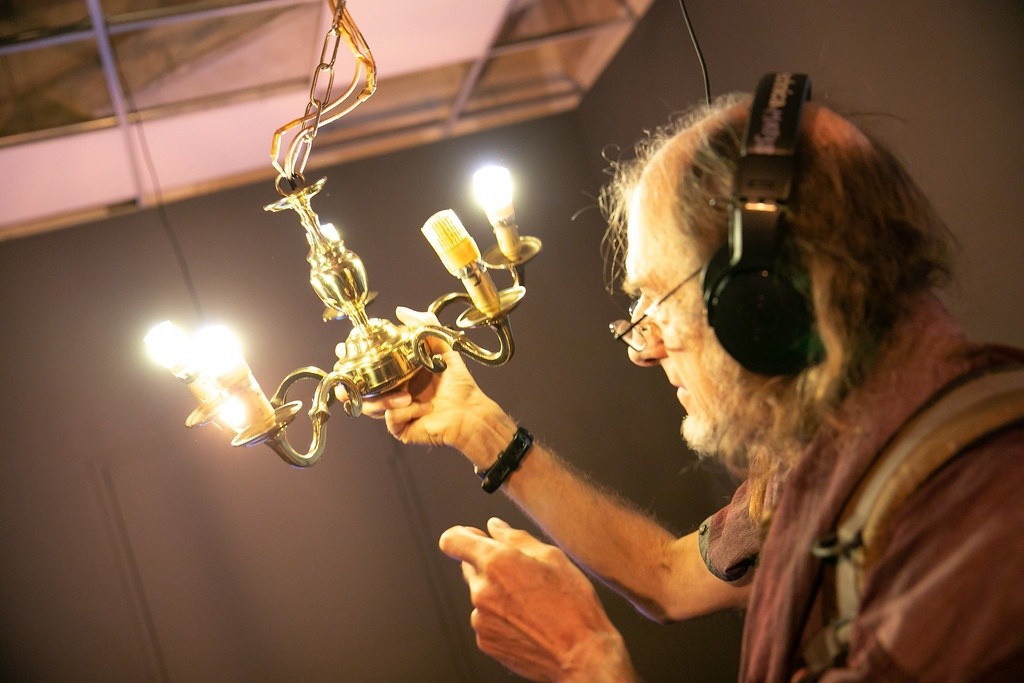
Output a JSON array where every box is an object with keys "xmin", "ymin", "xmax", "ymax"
[{"xmin": 139, "ymin": 0, "xmax": 544, "ymax": 470}]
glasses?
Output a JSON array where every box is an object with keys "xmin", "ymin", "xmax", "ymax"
[{"xmin": 610, "ymin": 268, "xmax": 702, "ymax": 351}]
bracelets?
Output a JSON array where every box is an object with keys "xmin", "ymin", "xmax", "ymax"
[{"xmin": 474, "ymin": 427, "xmax": 535, "ymax": 494}]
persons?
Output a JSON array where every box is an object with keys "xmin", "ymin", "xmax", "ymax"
[{"xmin": 334, "ymin": 70, "xmax": 1024, "ymax": 683}]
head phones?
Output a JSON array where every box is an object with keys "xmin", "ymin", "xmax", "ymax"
[{"xmin": 701, "ymin": 71, "xmax": 821, "ymax": 381}]
